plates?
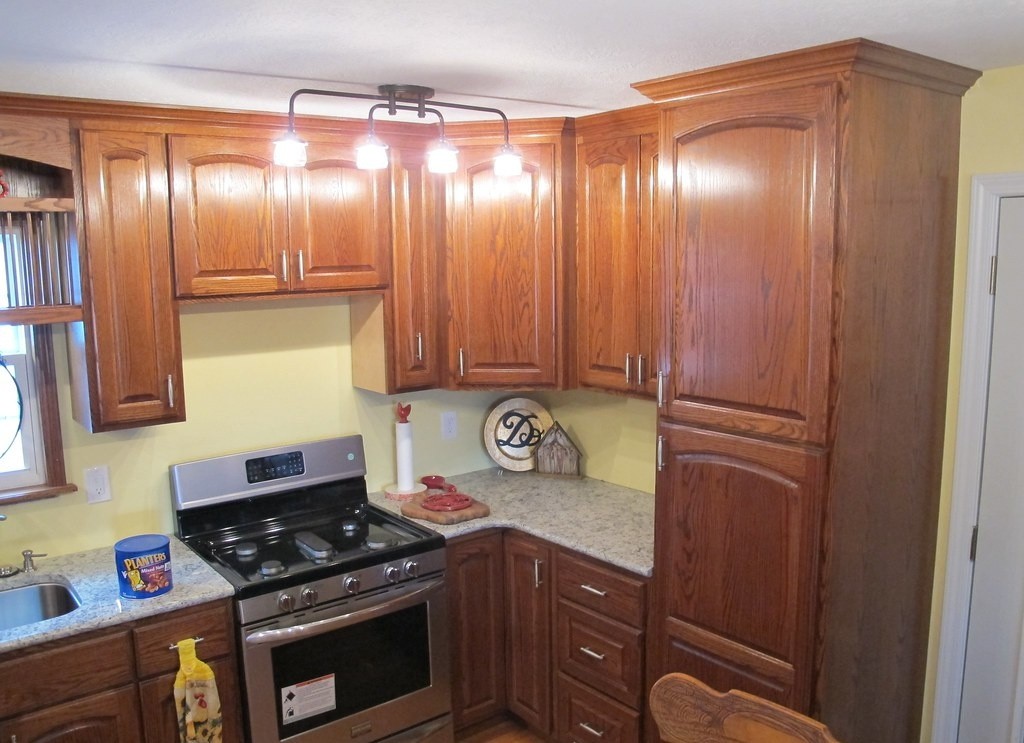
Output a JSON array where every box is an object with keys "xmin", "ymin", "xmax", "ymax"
[{"xmin": 484, "ymin": 397, "xmax": 554, "ymax": 472}]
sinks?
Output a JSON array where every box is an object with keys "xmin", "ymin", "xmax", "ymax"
[{"xmin": 0, "ymin": 573, "xmax": 83, "ymax": 632}]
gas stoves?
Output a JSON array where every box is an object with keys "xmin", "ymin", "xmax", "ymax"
[{"xmin": 168, "ymin": 433, "xmax": 448, "ymax": 626}]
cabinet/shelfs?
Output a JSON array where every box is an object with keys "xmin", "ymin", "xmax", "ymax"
[
  {"xmin": 445, "ymin": 528, "xmax": 653, "ymax": 743},
  {"xmin": 0, "ymin": 595, "xmax": 247, "ymax": 743},
  {"xmin": 0, "ymin": 91, "xmax": 663, "ymax": 435},
  {"xmin": 629, "ymin": 37, "xmax": 987, "ymax": 743}
]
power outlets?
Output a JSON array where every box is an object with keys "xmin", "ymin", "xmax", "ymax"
[{"xmin": 82, "ymin": 464, "xmax": 112, "ymax": 505}]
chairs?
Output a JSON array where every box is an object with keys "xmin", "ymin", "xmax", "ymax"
[{"xmin": 648, "ymin": 673, "xmax": 840, "ymax": 743}]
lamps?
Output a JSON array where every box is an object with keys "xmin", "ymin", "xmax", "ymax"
[{"xmin": 271, "ymin": 84, "xmax": 523, "ymax": 178}]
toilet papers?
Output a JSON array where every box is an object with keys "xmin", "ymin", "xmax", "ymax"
[{"xmin": 395, "ymin": 420, "xmax": 413, "ymax": 491}]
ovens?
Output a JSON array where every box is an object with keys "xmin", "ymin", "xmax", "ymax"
[{"xmin": 237, "ymin": 570, "xmax": 454, "ymax": 743}]
